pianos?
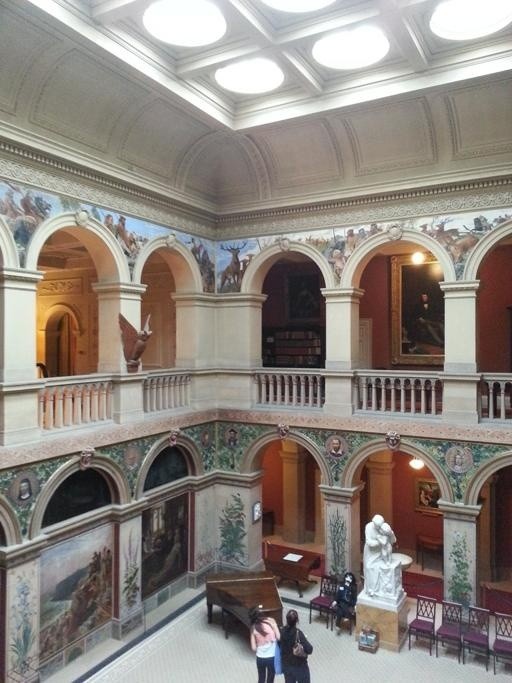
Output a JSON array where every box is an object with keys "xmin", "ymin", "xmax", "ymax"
[{"xmin": 205, "ymin": 574, "xmax": 281, "ymax": 642}]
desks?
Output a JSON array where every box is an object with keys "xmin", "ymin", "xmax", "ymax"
[
  {"xmin": 261, "ymin": 540, "xmax": 321, "ymax": 598},
  {"xmin": 414, "ymin": 534, "xmax": 443, "ymax": 573}
]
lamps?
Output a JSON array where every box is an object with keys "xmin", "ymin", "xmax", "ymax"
[{"xmin": 409, "ymin": 456, "xmax": 425, "ymax": 469}]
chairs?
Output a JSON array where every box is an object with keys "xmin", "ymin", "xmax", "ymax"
[
  {"xmin": 330, "ymin": 583, "xmax": 356, "ymax": 635},
  {"xmin": 409, "ymin": 592, "xmax": 437, "ymax": 656},
  {"xmin": 309, "ymin": 573, "xmax": 337, "ymax": 629},
  {"xmin": 435, "ymin": 599, "xmax": 463, "ymax": 662},
  {"xmin": 463, "ymin": 604, "xmax": 491, "ymax": 671},
  {"xmin": 492, "ymin": 610, "xmax": 512, "ymax": 675}
]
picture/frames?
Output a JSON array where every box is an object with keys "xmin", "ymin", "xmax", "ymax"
[
  {"xmin": 388, "ymin": 251, "xmax": 446, "ymax": 366},
  {"xmin": 414, "ymin": 476, "xmax": 443, "ymax": 517}
]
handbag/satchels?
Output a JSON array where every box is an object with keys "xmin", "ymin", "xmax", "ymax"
[{"xmin": 292, "ymin": 639, "xmax": 308, "ymax": 657}]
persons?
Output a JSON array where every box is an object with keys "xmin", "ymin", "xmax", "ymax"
[
  {"xmin": 361, "ymin": 514, "xmax": 405, "ymax": 606},
  {"xmin": 410, "ymin": 292, "xmax": 444, "ymax": 347},
  {"xmin": 278, "ymin": 610, "xmax": 313, "ymax": 683},
  {"xmin": 247, "ymin": 607, "xmax": 280, "ymax": 683},
  {"xmin": 330, "ymin": 572, "xmax": 358, "ymax": 635}
]
